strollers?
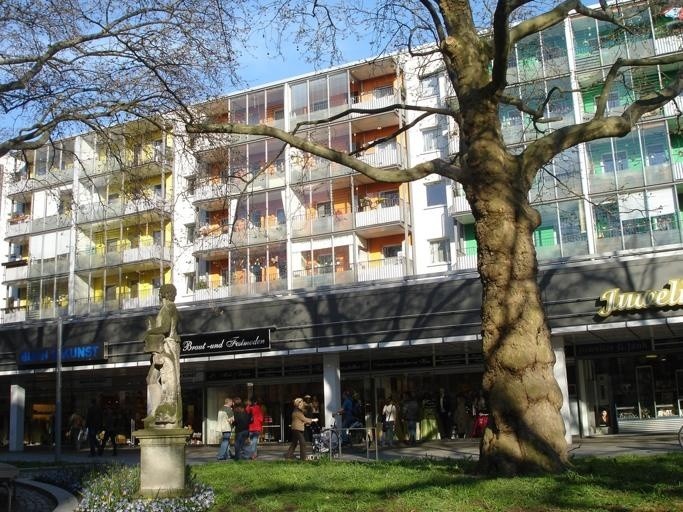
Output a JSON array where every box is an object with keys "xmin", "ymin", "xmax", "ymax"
[{"xmin": 307, "ymin": 422, "xmax": 339, "ymax": 460}]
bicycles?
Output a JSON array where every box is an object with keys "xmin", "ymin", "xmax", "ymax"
[
  {"xmin": 320, "ymin": 413, "xmax": 370, "ymax": 453},
  {"xmin": 678, "ymin": 425, "xmax": 683, "ymax": 448}
]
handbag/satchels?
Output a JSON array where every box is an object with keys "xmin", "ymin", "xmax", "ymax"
[{"xmin": 383, "ymin": 423, "xmax": 388, "ymax": 432}]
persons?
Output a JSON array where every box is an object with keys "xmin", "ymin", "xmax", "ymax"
[
  {"xmin": 335, "ymin": 391, "xmax": 356, "ymax": 446},
  {"xmin": 67, "ymin": 396, "xmax": 118, "ymax": 457},
  {"xmin": 285, "ymin": 395, "xmax": 319, "ymax": 460},
  {"xmin": 473, "ymin": 388, "xmax": 491, "ymax": 438},
  {"xmin": 217, "ymin": 397, "xmax": 265, "ymax": 460},
  {"xmin": 435, "ymin": 387, "xmax": 456, "ymax": 441},
  {"xmin": 353, "ymin": 392, "xmax": 368, "ymax": 426},
  {"xmin": 379, "ymin": 391, "xmax": 433, "ymax": 447},
  {"xmin": 139, "ymin": 283, "xmax": 183, "ymax": 423}
]
